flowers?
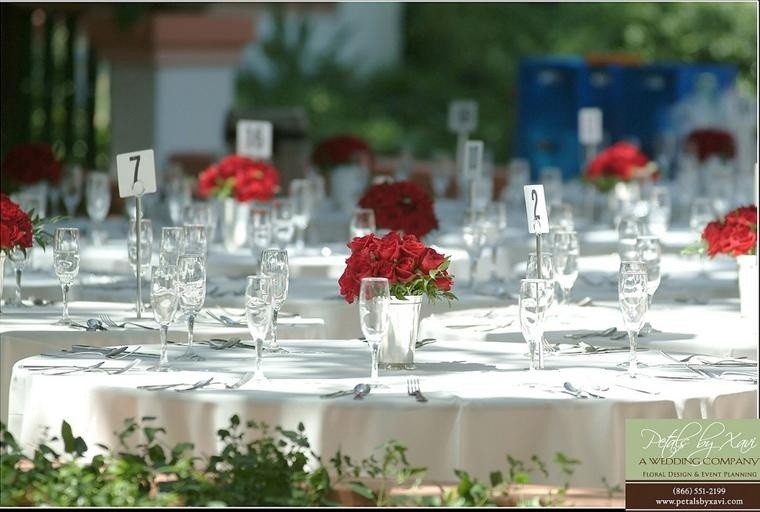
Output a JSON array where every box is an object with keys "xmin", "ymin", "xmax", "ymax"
[
  {"xmin": 683, "ymin": 129, "xmax": 736, "ymax": 164},
  {"xmin": 358, "ymin": 181, "xmax": 440, "ymax": 239},
  {"xmin": 310, "ymin": 136, "xmax": 376, "ymax": 173},
  {"xmin": 585, "ymin": 142, "xmax": 660, "ymax": 192},
  {"xmin": 194, "ymin": 155, "xmax": 279, "ymax": 201}
]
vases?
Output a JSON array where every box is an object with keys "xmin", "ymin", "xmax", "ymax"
[{"xmin": 329, "ymin": 166, "xmax": 370, "ymax": 217}]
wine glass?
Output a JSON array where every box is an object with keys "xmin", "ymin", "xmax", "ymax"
[
  {"xmin": 359, "ymin": 277, "xmax": 390, "ymax": 388},
  {"xmin": 182, "ymin": 178, "xmax": 721, "ymax": 288},
  {"xmin": 518, "ymin": 229, "xmax": 664, "ymax": 385},
  {"xmin": 258, "ymin": 249, "xmax": 291, "ymax": 355},
  {"xmin": 244, "ymin": 274, "xmax": 278, "ymax": 388},
  {"xmin": 52, "ymin": 218, "xmax": 208, "ymax": 374}
]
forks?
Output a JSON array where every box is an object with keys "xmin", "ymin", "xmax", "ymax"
[
  {"xmin": 405, "ymin": 379, "xmax": 429, "ymax": 403},
  {"xmin": 205, "ymin": 336, "xmax": 240, "ymax": 351}
]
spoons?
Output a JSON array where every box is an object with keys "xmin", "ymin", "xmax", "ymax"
[
  {"xmin": 352, "ymin": 383, "xmax": 371, "ymax": 400},
  {"xmin": 560, "ymin": 380, "xmax": 606, "ymax": 400}
]
